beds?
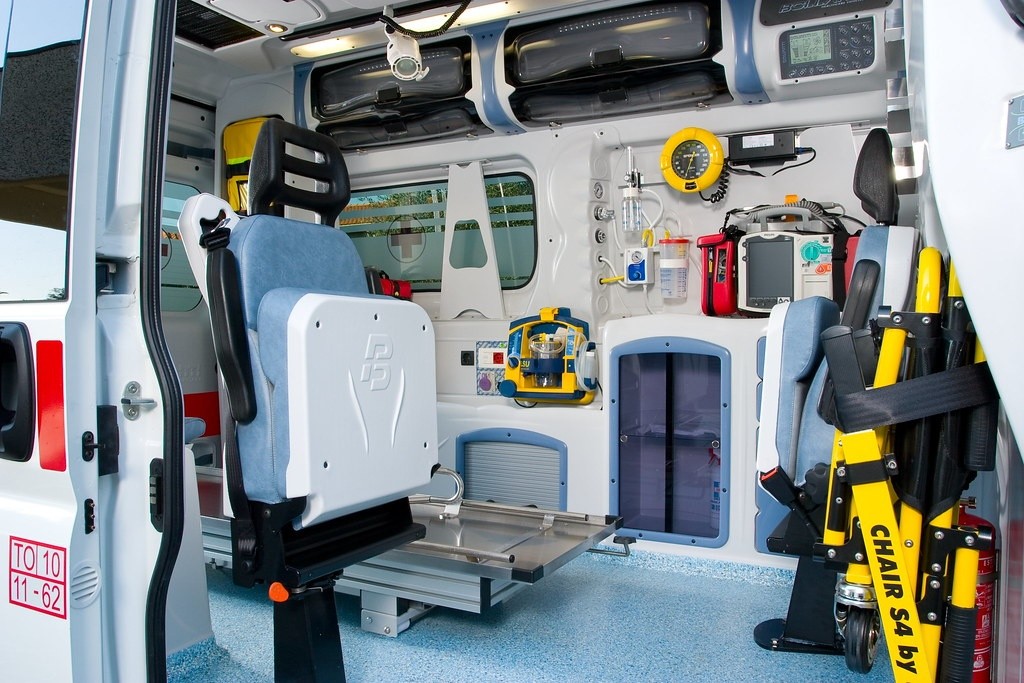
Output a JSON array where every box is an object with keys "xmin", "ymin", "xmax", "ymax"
[{"xmin": 189, "ymin": 439, "xmax": 638, "ymax": 637}]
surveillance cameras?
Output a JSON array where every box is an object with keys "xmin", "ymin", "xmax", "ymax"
[{"xmin": 386, "ymin": 35, "xmax": 423, "ymax": 81}]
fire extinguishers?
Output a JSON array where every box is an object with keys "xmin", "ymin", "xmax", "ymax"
[{"xmin": 956, "ymin": 495, "xmax": 998, "ymax": 683}]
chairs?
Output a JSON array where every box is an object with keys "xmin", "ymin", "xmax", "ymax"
[
  {"xmin": 754, "ymin": 128, "xmax": 922, "ymax": 657},
  {"xmin": 177, "ymin": 118, "xmax": 442, "ymax": 682}
]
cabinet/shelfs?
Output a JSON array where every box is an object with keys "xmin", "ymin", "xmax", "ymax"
[
  {"xmin": 294, "ymin": 0, "xmax": 770, "ymax": 157},
  {"xmin": 608, "ymin": 337, "xmax": 732, "ymax": 548}
]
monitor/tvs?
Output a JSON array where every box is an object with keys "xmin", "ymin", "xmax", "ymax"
[{"xmin": 747, "ymin": 242, "xmax": 794, "ymax": 298}]
